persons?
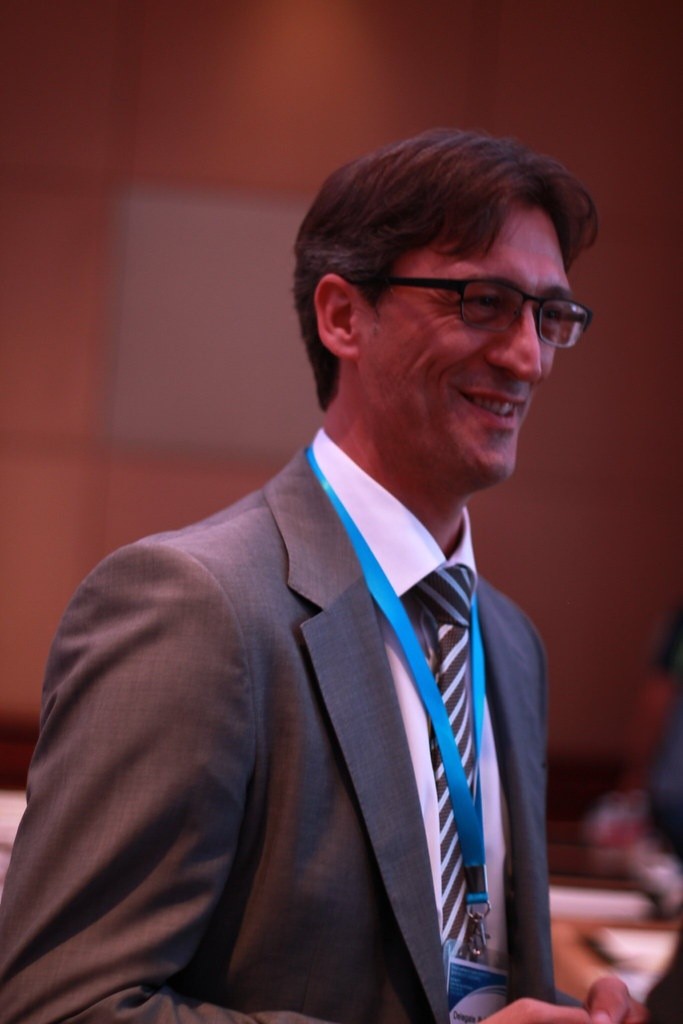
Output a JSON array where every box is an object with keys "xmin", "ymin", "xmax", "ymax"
[
  {"xmin": 587, "ymin": 604, "xmax": 683, "ymax": 1024},
  {"xmin": 1, "ymin": 131, "xmax": 649, "ymax": 1024}
]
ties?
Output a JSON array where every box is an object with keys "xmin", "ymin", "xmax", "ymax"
[{"xmin": 403, "ymin": 564, "xmax": 482, "ymax": 961}]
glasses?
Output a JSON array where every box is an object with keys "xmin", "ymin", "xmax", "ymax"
[{"xmin": 351, "ymin": 268, "xmax": 592, "ymax": 348}]
